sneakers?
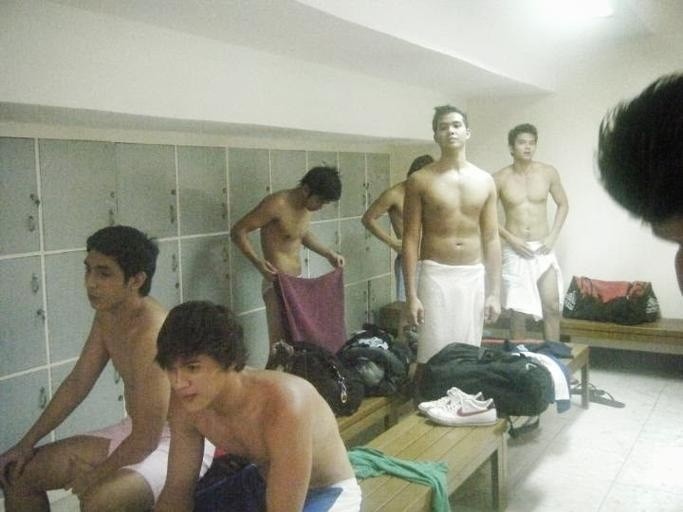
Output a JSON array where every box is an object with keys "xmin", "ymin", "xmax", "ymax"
[{"xmin": 420, "ymin": 387, "xmax": 497, "ymax": 426}]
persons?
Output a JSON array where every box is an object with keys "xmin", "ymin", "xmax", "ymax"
[
  {"xmin": 492, "ymin": 122, "xmax": 569, "ymax": 341},
  {"xmin": 226, "ymin": 163, "xmax": 346, "ymax": 371},
  {"xmin": 401, "ymin": 104, "xmax": 503, "ymax": 359},
  {"xmin": 148, "ymin": 300, "xmax": 363, "ymax": 511},
  {"xmin": 593, "ymin": 69, "xmax": 683, "ymax": 299},
  {"xmin": 0, "ymin": 224, "xmax": 214, "ymax": 512},
  {"xmin": 361, "ymin": 153, "xmax": 436, "ymax": 306}
]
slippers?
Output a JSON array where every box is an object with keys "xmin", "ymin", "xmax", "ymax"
[{"xmin": 570, "ymin": 379, "xmax": 624, "ymax": 408}]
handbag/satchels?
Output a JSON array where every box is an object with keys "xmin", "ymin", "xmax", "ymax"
[
  {"xmin": 266, "ymin": 340, "xmax": 365, "ymax": 416},
  {"xmin": 564, "ymin": 275, "xmax": 659, "ymax": 326},
  {"xmin": 197, "ymin": 452, "xmax": 265, "ymax": 512},
  {"xmin": 418, "ymin": 342, "xmax": 552, "ymax": 414},
  {"xmin": 337, "ymin": 322, "xmax": 413, "ymax": 397}
]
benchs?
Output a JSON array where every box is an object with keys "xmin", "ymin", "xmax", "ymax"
[
  {"xmin": 348, "ymin": 340, "xmax": 590, "ymax": 512},
  {"xmin": 333, "ymin": 357, "xmax": 422, "ymax": 454},
  {"xmin": 375, "ymin": 299, "xmax": 683, "ymax": 357}
]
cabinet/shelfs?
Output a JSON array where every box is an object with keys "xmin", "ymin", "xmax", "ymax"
[{"xmin": 1, "ymin": 135, "xmax": 392, "ymax": 504}]
